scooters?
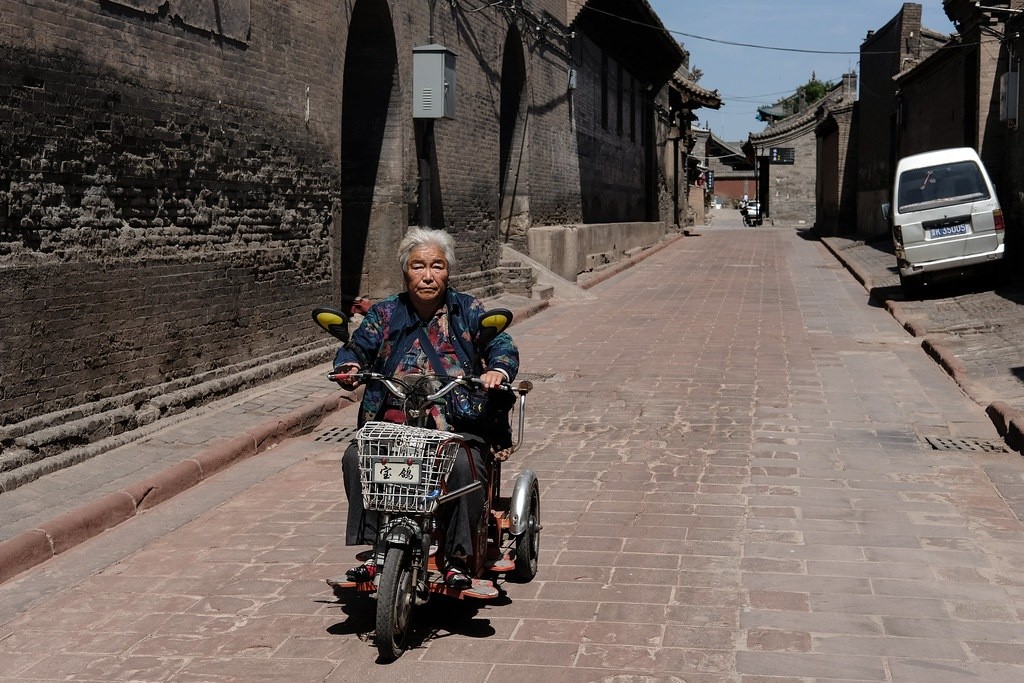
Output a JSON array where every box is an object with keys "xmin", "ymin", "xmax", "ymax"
[{"xmin": 310, "ymin": 304, "xmax": 543, "ymax": 662}]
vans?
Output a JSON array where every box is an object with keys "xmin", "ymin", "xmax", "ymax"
[{"xmin": 877, "ymin": 146, "xmax": 1007, "ymax": 292}]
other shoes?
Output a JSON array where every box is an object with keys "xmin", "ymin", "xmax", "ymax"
[
  {"xmin": 345, "ymin": 553, "xmax": 386, "ymax": 582},
  {"xmin": 435, "ymin": 552, "xmax": 472, "ymax": 590}
]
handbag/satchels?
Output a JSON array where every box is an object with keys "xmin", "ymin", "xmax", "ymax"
[{"xmin": 451, "ymin": 385, "xmax": 487, "ymax": 430}]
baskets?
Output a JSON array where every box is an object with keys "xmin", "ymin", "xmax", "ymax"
[{"xmin": 355, "ymin": 420, "xmax": 464, "ymax": 513}]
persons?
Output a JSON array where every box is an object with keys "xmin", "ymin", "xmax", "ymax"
[
  {"xmin": 742, "ymin": 193, "xmax": 748, "ymax": 206},
  {"xmin": 332, "ymin": 227, "xmax": 519, "ymax": 590}
]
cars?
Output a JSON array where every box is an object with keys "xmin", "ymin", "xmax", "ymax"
[{"xmin": 746, "ymin": 199, "xmax": 761, "ymax": 217}]
